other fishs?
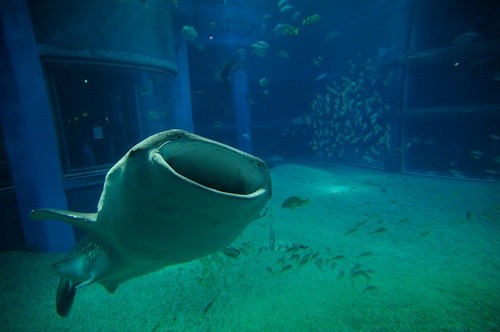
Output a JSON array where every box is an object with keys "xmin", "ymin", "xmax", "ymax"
[
  {"xmin": 29, "ymin": 127, "xmax": 273, "ymax": 318},
  {"xmin": 147, "ymin": 0, "xmax": 471, "ymax": 332}
]
